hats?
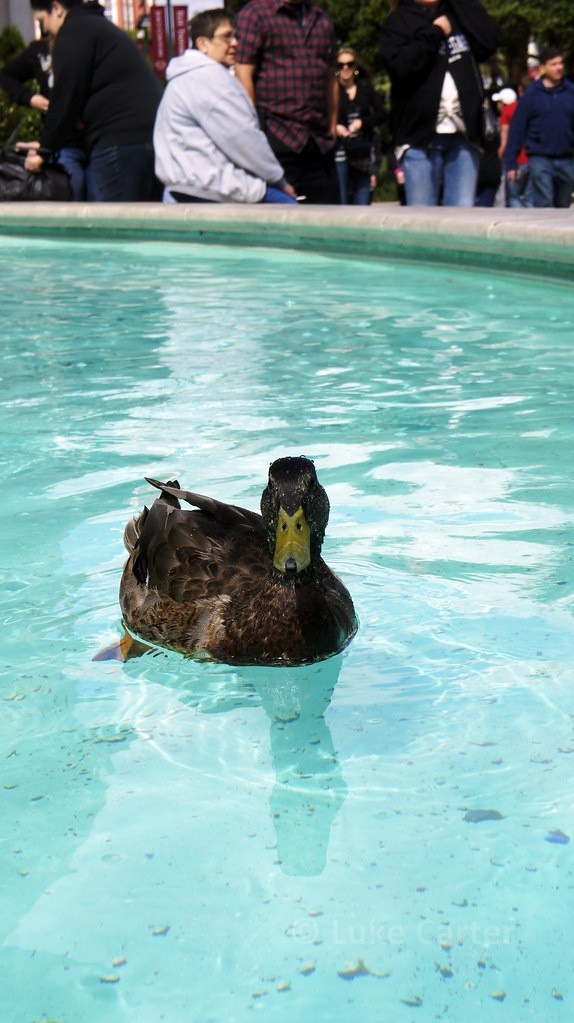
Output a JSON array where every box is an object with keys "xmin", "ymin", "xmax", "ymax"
[{"xmin": 491, "ymin": 88, "xmax": 517, "ymax": 106}]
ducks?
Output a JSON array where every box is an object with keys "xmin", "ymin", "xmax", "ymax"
[{"xmin": 92, "ymin": 455, "xmax": 358, "ymax": 666}]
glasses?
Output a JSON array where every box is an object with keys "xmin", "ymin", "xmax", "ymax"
[
  {"xmin": 210, "ymin": 31, "xmax": 242, "ymax": 43},
  {"xmin": 337, "ymin": 61, "xmax": 356, "ymax": 70}
]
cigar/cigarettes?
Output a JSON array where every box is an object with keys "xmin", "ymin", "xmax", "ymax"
[{"xmin": 296, "ymin": 196, "xmax": 305, "ymax": 201}]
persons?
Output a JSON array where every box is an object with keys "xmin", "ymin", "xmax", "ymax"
[
  {"xmin": 324, "ymin": 48, "xmax": 375, "ymax": 207},
  {"xmin": 0, "ymin": 27, "xmax": 59, "ymax": 130},
  {"xmin": 481, "ymin": 56, "xmax": 550, "ymax": 206},
  {"xmin": 17, "ymin": 1, "xmax": 162, "ymax": 204},
  {"xmin": 380, "ymin": 1, "xmax": 483, "ymax": 209},
  {"xmin": 136, "ymin": 0, "xmax": 150, "ymax": 42},
  {"xmin": 151, "ymin": 9, "xmax": 298, "ymax": 204},
  {"xmin": 237, "ymin": 1, "xmax": 342, "ymax": 204},
  {"xmin": 503, "ymin": 51, "xmax": 574, "ymax": 209}
]
